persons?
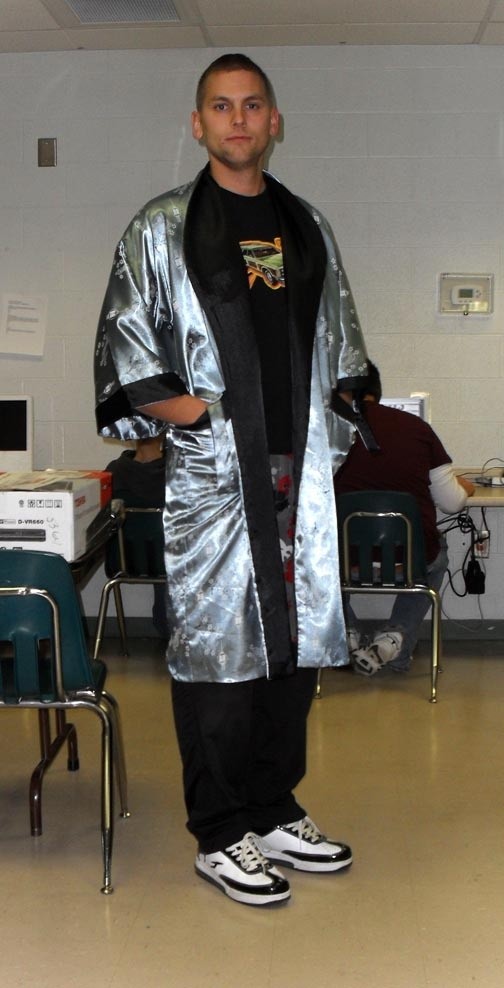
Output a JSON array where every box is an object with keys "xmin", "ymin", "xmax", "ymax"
[
  {"xmin": 332, "ymin": 358, "xmax": 477, "ymax": 675},
  {"xmin": 93, "ymin": 52, "xmax": 373, "ymax": 906},
  {"xmin": 103, "ymin": 432, "xmax": 168, "ymax": 639}
]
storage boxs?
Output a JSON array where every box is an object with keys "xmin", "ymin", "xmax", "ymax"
[{"xmin": 0, "ymin": 466, "xmax": 113, "ymax": 562}]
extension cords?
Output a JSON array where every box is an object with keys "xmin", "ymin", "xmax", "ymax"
[{"xmin": 490, "ymin": 476, "xmax": 504, "ymax": 486}]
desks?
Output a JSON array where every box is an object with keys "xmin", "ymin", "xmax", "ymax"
[
  {"xmin": 26, "ymin": 519, "xmax": 117, "ymax": 838},
  {"xmin": 454, "ymin": 470, "xmax": 504, "ymax": 507}
]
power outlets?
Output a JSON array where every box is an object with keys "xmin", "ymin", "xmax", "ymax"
[{"xmin": 474, "ymin": 530, "xmax": 489, "ymax": 559}]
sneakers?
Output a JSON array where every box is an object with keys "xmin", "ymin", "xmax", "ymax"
[
  {"xmin": 343, "ymin": 626, "xmax": 359, "ymax": 651},
  {"xmin": 258, "ymin": 815, "xmax": 353, "ymax": 874},
  {"xmin": 194, "ymin": 832, "xmax": 291, "ymax": 905},
  {"xmin": 350, "ymin": 631, "xmax": 403, "ymax": 676}
]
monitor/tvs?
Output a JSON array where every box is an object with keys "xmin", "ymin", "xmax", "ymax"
[{"xmin": 1, "ymin": 394, "xmax": 34, "ymax": 475}]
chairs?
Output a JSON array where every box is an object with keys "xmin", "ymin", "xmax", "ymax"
[
  {"xmin": 313, "ymin": 490, "xmax": 444, "ymax": 704},
  {"xmin": 90, "ymin": 489, "xmax": 169, "ymax": 660},
  {"xmin": 0, "ymin": 550, "xmax": 132, "ymax": 895}
]
